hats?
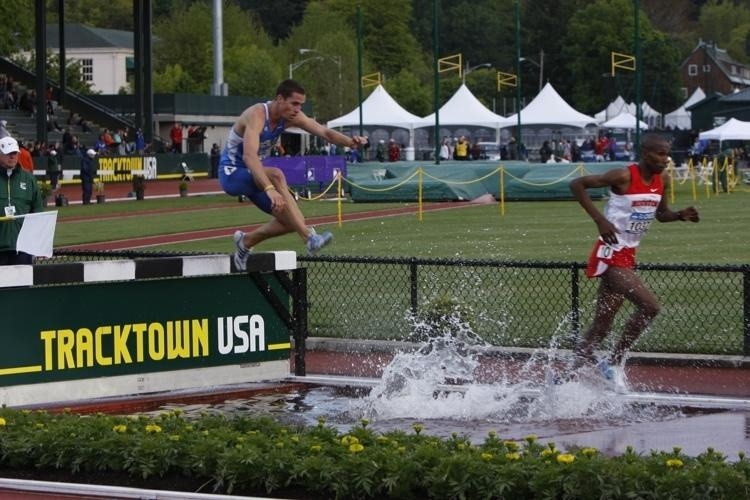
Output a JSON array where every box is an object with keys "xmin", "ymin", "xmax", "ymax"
[
  {"xmin": 86, "ymin": 149, "xmax": 97, "ymax": 156},
  {"xmin": 0, "ymin": 137, "xmax": 21, "ymax": 156}
]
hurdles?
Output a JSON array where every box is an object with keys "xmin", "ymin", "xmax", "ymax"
[{"xmin": 0, "ymin": 250, "xmax": 308, "ymax": 378}]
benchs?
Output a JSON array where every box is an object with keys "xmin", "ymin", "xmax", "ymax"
[{"xmin": 1, "ymin": 91, "xmax": 107, "ymax": 156}]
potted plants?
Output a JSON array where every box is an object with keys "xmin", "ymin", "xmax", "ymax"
[
  {"xmin": 132, "ymin": 174, "xmax": 146, "ymax": 200},
  {"xmin": 95, "ymin": 182, "xmax": 105, "ymax": 203},
  {"xmin": 36, "ymin": 181, "xmax": 52, "ymax": 208},
  {"xmin": 178, "ymin": 179, "xmax": 189, "ymax": 197}
]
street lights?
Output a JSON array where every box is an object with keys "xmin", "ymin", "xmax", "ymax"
[
  {"xmin": 299, "ymin": 47, "xmax": 345, "ymax": 118},
  {"xmin": 520, "ymin": 49, "xmax": 545, "ymax": 95},
  {"xmin": 288, "ymin": 55, "xmax": 325, "ymax": 78},
  {"xmin": 462, "ymin": 62, "xmax": 492, "ymax": 86}
]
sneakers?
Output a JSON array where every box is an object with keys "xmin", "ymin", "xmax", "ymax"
[
  {"xmin": 232, "ymin": 229, "xmax": 254, "ymax": 273},
  {"xmin": 596, "ymin": 358, "xmax": 629, "ymax": 395},
  {"xmin": 305, "ymin": 229, "xmax": 333, "ymax": 257}
]
